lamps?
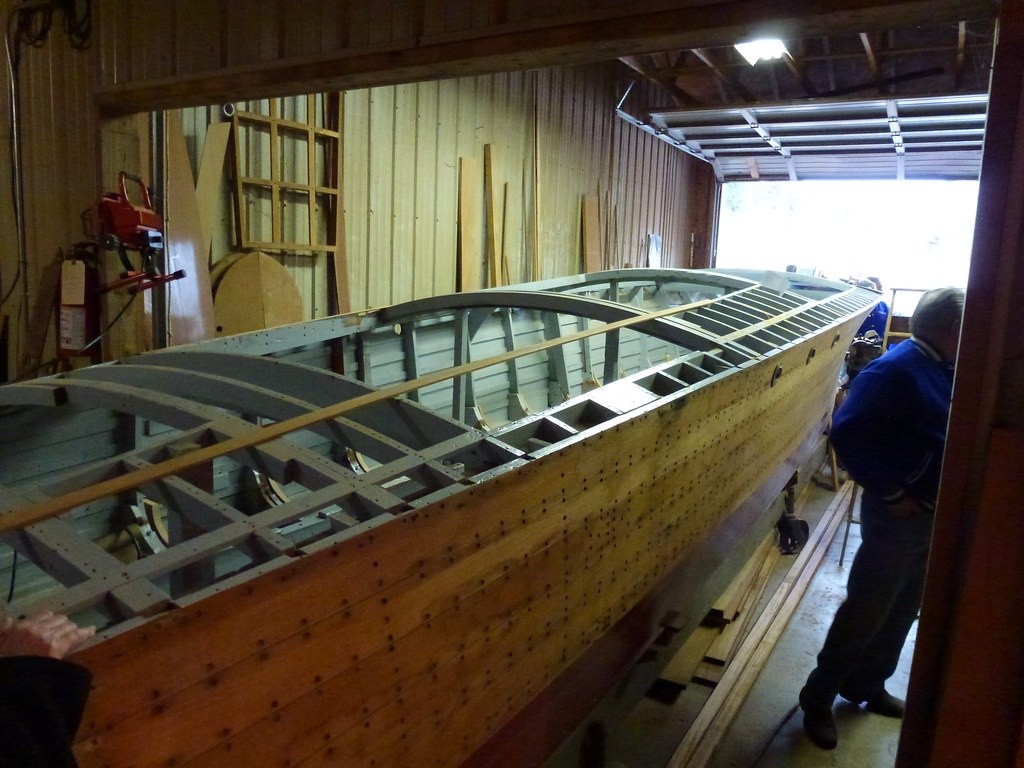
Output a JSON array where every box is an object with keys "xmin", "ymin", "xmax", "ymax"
[{"xmin": 733, "ymin": 38, "xmax": 795, "ymax": 66}]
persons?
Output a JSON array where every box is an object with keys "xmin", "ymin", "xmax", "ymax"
[{"xmin": 800, "ymin": 286, "xmax": 966, "ymax": 750}]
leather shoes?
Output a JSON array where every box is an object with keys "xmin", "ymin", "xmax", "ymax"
[
  {"xmin": 838, "ymin": 681, "xmax": 907, "ymax": 718},
  {"xmin": 799, "ymin": 683, "xmax": 838, "ymax": 750}
]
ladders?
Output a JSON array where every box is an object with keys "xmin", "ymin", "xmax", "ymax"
[{"xmin": 835, "ymin": 287, "xmax": 938, "ymax": 566}]
